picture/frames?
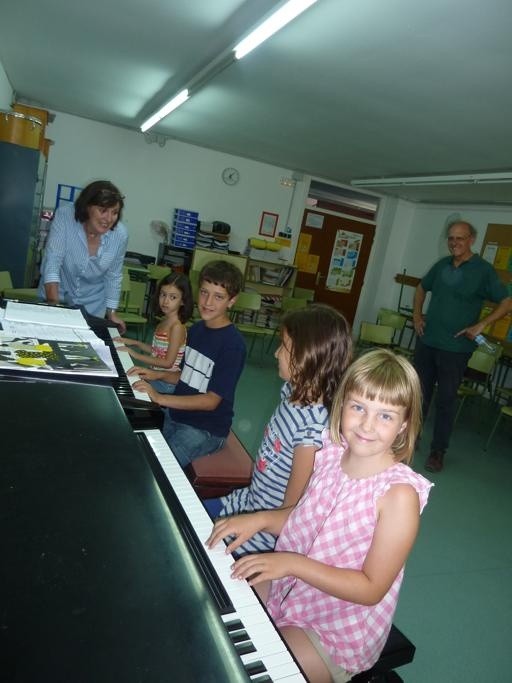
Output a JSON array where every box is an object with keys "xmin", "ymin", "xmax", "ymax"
[{"xmin": 258, "ymin": 210, "xmax": 279, "ymax": 238}]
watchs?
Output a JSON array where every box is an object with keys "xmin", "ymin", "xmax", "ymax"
[{"xmin": 106, "ymin": 308, "xmax": 117, "ymax": 314}]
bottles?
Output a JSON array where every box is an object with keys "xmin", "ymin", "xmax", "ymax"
[{"xmin": 473, "ymin": 333, "xmax": 496, "ymax": 354}]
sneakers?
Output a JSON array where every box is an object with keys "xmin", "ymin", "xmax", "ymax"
[
  {"xmin": 425, "ymin": 450, "xmax": 447, "ymax": 473},
  {"xmin": 414, "ymin": 440, "xmax": 420, "ymax": 450}
]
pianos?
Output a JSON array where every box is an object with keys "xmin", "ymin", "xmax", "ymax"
[
  {"xmin": 0, "ymin": 290, "xmax": 164, "ymax": 428},
  {"xmin": 1, "ymin": 374, "xmax": 308, "ymax": 683}
]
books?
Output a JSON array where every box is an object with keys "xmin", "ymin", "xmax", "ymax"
[
  {"xmin": 225, "ymin": 307, "xmax": 253, "ymax": 328},
  {"xmin": 0, "ymin": 299, "xmax": 118, "ymax": 377},
  {"xmin": 244, "ymin": 246, "xmax": 289, "ymax": 266},
  {"xmin": 196, "ymin": 221, "xmax": 229, "ymax": 254},
  {"xmin": 254, "ymin": 302, "xmax": 284, "ymax": 330},
  {"xmin": 248, "ymin": 265, "xmax": 294, "ymax": 288}
]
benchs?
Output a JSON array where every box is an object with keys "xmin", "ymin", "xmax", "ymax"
[
  {"xmin": 338, "ymin": 622, "xmax": 416, "ymax": 682},
  {"xmin": 187, "ymin": 424, "xmax": 256, "ymax": 499}
]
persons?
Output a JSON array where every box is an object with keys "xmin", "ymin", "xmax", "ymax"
[
  {"xmin": 410, "ymin": 221, "xmax": 511, "ymax": 473},
  {"xmin": 39, "ymin": 181, "xmax": 127, "ymax": 340},
  {"xmin": 112, "ymin": 271, "xmax": 192, "ymax": 377},
  {"xmin": 203, "ymin": 349, "xmax": 433, "ymax": 683},
  {"xmin": 203, "ymin": 304, "xmax": 353, "ymax": 560},
  {"xmin": 124, "ymin": 260, "xmax": 249, "ymax": 477}
]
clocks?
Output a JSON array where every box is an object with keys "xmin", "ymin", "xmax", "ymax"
[{"xmin": 222, "ymin": 167, "xmax": 240, "ymax": 186}]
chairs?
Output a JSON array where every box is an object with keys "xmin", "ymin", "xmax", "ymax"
[
  {"xmin": 233, "ymin": 293, "xmax": 268, "ymax": 362},
  {"xmin": 110, "ymin": 271, "xmax": 147, "ymax": 340},
  {"xmin": 460, "ymin": 339, "xmax": 505, "ymax": 408},
  {"xmin": 487, "ymin": 385, "xmax": 512, "ymax": 407},
  {"xmin": 484, "ymin": 405, "xmax": 512, "ymax": 447},
  {"xmin": 437, "ymin": 349, "xmax": 495, "ymax": 424},
  {"xmin": 267, "ymin": 297, "xmax": 319, "ymax": 356},
  {"xmin": 376, "ymin": 309, "xmax": 407, "ymax": 349},
  {"xmin": 144, "ymin": 263, "xmax": 195, "ymax": 338},
  {"xmin": 354, "ymin": 320, "xmax": 391, "ymax": 372}
]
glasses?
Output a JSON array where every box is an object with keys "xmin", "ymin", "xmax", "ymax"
[
  {"xmin": 101, "ymin": 187, "xmax": 127, "ymax": 200},
  {"xmin": 447, "ymin": 237, "xmax": 463, "ymax": 241}
]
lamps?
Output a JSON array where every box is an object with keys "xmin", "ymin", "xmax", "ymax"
[{"xmin": 138, "ymin": 0, "xmax": 318, "ymax": 134}]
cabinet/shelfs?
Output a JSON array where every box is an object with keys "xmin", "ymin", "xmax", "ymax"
[
  {"xmin": 142, "ymin": 241, "xmax": 193, "ymax": 326},
  {"xmin": 233, "ymin": 256, "xmax": 299, "ymax": 335},
  {"xmin": 118, "ymin": 251, "xmax": 153, "ymax": 317},
  {"xmin": 26, "ymin": 145, "xmax": 49, "ymax": 288},
  {"xmin": 189, "ymin": 248, "xmax": 248, "ymax": 333}
]
